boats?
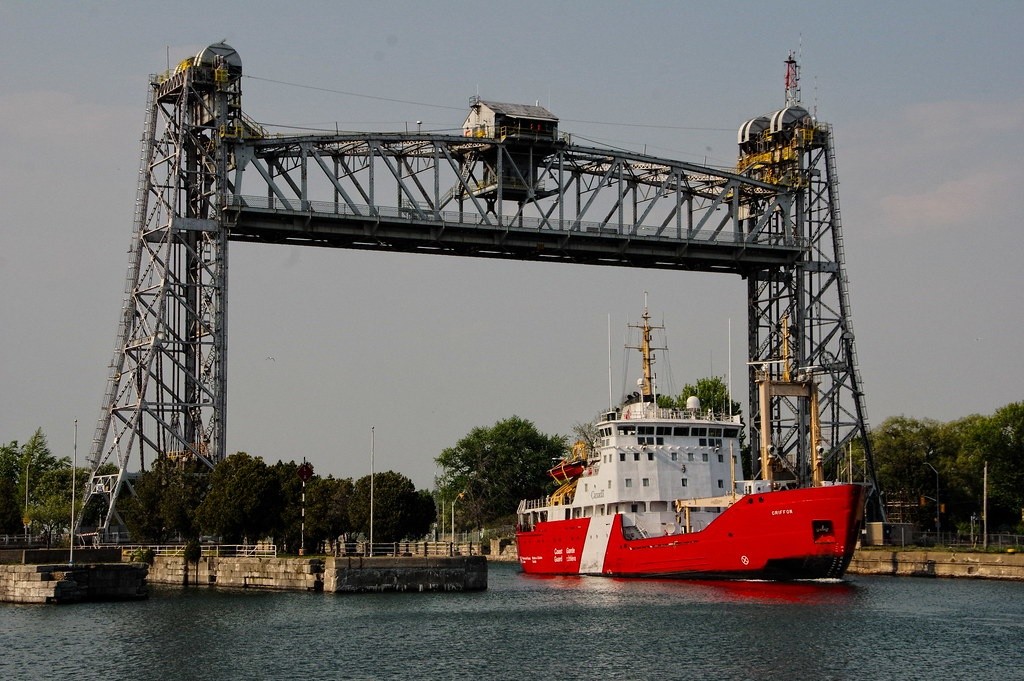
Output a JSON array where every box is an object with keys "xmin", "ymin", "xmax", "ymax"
[{"xmin": 517, "ymin": 289, "xmax": 873, "ymax": 585}]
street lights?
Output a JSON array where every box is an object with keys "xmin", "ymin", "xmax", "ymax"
[
  {"xmin": 24, "ymin": 456, "xmax": 37, "ymax": 542},
  {"xmin": 923, "ymin": 461, "xmax": 940, "ymax": 544},
  {"xmin": 451, "ymin": 492, "xmax": 464, "ymax": 552}
]
